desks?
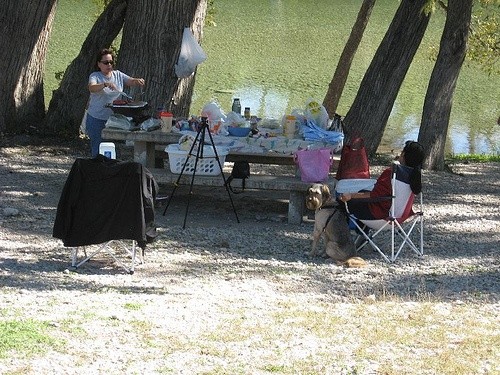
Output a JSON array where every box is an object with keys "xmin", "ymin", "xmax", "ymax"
[{"xmin": 101, "ymin": 128, "xmax": 341, "ymax": 176}]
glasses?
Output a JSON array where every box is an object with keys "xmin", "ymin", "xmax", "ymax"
[{"xmin": 99, "ymin": 60, "xmax": 113, "ymax": 65}]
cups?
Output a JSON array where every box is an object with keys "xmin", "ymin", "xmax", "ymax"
[{"xmin": 285, "ymin": 115, "xmax": 296, "ymax": 139}]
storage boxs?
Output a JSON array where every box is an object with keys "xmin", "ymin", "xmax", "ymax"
[
  {"xmin": 335, "ymin": 178, "xmax": 377, "ymax": 229},
  {"xmin": 165, "ymin": 144, "xmax": 230, "ymax": 176}
]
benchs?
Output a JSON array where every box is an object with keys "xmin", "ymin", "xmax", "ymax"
[{"xmin": 146, "ymin": 168, "xmax": 338, "ymax": 225}]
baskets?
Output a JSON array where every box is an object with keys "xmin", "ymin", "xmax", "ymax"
[{"xmin": 164, "ymin": 144, "xmax": 230, "ymax": 176}]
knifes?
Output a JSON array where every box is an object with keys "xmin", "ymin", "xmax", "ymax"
[{"xmin": 107, "ymin": 81, "xmax": 133, "ymax": 99}]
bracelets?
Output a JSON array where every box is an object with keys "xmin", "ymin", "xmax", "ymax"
[{"xmin": 103, "ymin": 82, "xmax": 107, "ymax": 87}]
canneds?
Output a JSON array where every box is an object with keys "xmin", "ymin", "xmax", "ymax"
[{"xmin": 245, "ymin": 107, "xmax": 251, "ymax": 118}]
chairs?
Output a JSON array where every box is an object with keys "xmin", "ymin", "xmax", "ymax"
[
  {"xmin": 52, "ymin": 154, "xmax": 154, "ymax": 275},
  {"xmin": 349, "ymin": 173, "xmax": 424, "ymax": 264}
]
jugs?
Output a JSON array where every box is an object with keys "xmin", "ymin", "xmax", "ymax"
[{"xmin": 99, "ymin": 142, "xmax": 116, "ymax": 159}]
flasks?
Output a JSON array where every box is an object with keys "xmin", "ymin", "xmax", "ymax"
[
  {"xmin": 244, "ymin": 107, "xmax": 251, "ymax": 120},
  {"xmin": 232, "ymin": 98, "xmax": 241, "ymax": 114}
]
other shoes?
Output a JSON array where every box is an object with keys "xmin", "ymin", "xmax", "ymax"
[{"xmin": 356, "ymin": 233, "xmax": 372, "ymax": 249}]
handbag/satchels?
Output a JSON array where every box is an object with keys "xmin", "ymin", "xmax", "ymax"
[
  {"xmin": 174, "ymin": 28, "xmax": 206, "ymax": 78},
  {"xmin": 227, "ymin": 160, "xmax": 250, "ymax": 194},
  {"xmin": 336, "ymin": 137, "xmax": 370, "ymax": 180},
  {"xmin": 294, "ymin": 148, "xmax": 334, "ymax": 182}
]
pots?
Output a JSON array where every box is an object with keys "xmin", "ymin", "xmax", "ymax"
[{"xmin": 105, "ymin": 96, "xmax": 150, "ymax": 115}]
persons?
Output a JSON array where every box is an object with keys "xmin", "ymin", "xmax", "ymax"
[
  {"xmin": 86, "ymin": 48, "xmax": 145, "ymax": 158},
  {"xmin": 335, "ymin": 140, "xmax": 426, "ymax": 229}
]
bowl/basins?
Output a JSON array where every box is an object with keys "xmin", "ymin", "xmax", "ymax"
[{"xmin": 227, "ymin": 125, "xmax": 252, "ymax": 137}]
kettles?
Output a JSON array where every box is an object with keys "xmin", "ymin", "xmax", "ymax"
[{"xmin": 327, "ymin": 112, "xmax": 342, "ymax": 132}]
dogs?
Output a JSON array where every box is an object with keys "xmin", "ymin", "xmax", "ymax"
[{"xmin": 304, "ymin": 182, "xmax": 370, "ymax": 267}]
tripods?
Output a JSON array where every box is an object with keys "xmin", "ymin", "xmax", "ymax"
[{"xmin": 163, "ymin": 117, "xmax": 240, "ymax": 229}]
